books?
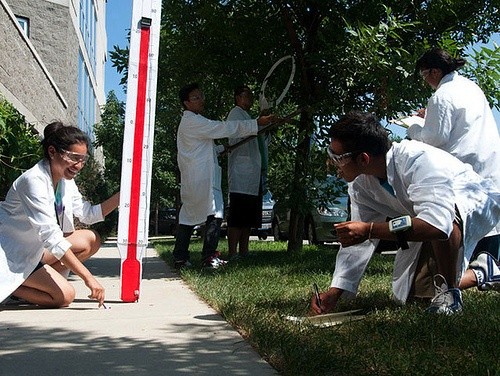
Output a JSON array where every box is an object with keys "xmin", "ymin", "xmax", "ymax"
[{"xmin": 281, "ymin": 309, "xmax": 366, "ymax": 328}]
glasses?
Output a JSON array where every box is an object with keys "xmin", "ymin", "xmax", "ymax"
[
  {"xmin": 326, "ymin": 145, "xmax": 355, "ymax": 167},
  {"xmin": 420, "ymin": 68, "xmax": 434, "ymax": 81},
  {"xmin": 58, "ymin": 147, "xmax": 90, "ymax": 165},
  {"xmin": 189, "ymin": 94, "xmax": 206, "ymax": 105}
]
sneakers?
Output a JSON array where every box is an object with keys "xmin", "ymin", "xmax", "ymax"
[
  {"xmin": 423, "ymin": 274, "xmax": 464, "ymax": 318},
  {"xmin": 467, "ymin": 252, "xmax": 500, "ymax": 290}
]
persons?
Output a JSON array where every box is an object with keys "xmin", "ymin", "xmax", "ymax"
[
  {"xmin": 225, "ymin": 85, "xmax": 269, "ymax": 261},
  {"xmin": 172, "ymin": 83, "xmax": 272, "ymax": 270},
  {"xmin": 407, "ymin": 48, "xmax": 500, "ymax": 259},
  {"xmin": 312, "ymin": 111, "xmax": 500, "ymax": 317},
  {"xmin": 0, "ymin": 122, "xmax": 120, "ymax": 306}
]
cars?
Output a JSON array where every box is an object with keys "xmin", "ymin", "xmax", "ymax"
[
  {"xmin": 271, "ymin": 196, "xmax": 349, "ymax": 245},
  {"xmin": 192, "ymin": 191, "xmax": 276, "ymax": 240},
  {"xmin": 149, "ymin": 209, "xmax": 178, "ymax": 237}
]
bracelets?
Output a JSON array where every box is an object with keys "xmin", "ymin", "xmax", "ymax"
[{"xmin": 369, "ymin": 222, "xmax": 374, "ymax": 238}]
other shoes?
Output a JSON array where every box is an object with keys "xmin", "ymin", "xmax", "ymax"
[
  {"xmin": 200, "ymin": 255, "xmax": 226, "ymax": 267},
  {"xmin": 174, "ymin": 259, "xmax": 194, "ymax": 270}
]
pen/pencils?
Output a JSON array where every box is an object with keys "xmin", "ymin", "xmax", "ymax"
[
  {"xmin": 101, "ymin": 301, "xmax": 108, "ymax": 310},
  {"xmin": 313, "ymin": 283, "xmax": 324, "ymax": 309}
]
